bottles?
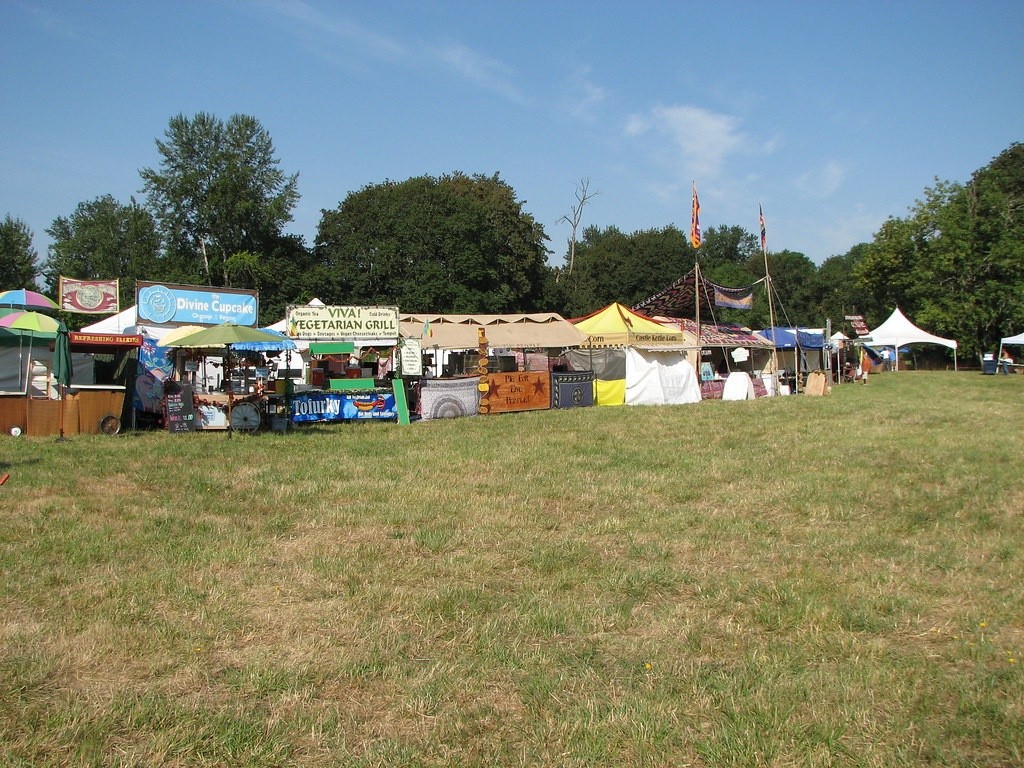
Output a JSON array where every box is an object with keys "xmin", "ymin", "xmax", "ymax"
[{"xmin": 312, "ymin": 368, "xmax": 324, "ymax": 386}]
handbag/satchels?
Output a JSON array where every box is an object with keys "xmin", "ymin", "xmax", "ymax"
[{"xmin": 862, "ymin": 372, "xmax": 867, "ymax": 379}]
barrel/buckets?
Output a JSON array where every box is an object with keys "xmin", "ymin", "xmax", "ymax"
[{"xmin": 983, "ymin": 360, "xmax": 996, "ymax": 374}]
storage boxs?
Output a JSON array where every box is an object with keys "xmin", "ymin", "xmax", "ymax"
[
  {"xmin": 312, "ymin": 368, "xmax": 324, "ymax": 385},
  {"xmin": 361, "ymin": 368, "xmax": 372, "ymax": 378},
  {"xmin": 345, "ymin": 366, "xmax": 361, "ymax": 378}
]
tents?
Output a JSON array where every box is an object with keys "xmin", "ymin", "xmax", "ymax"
[
  {"xmin": 78, "ymin": 301, "xmax": 234, "ymax": 433},
  {"xmin": 854, "ymin": 304, "xmax": 958, "ymax": 372},
  {"xmin": 249, "ymin": 263, "xmax": 853, "ymax": 427},
  {"xmin": 996, "ymin": 332, "xmax": 1024, "ymax": 374}
]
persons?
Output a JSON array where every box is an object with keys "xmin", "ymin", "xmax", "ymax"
[
  {"xmin": 880, "ymin": 346, "xmax": 896, "ymax": 372},
  {"xmin": 861, "ymin": 354, "xmax": 871, "ymax": 387},
  {"xmin": 1001, "ymin": 348, "xmax": 1009, "ymax": 376}
]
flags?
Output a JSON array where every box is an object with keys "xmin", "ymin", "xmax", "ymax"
[
  {"xmin": 690, "ymin": 178, "xmax": 702, "ymax": 250},
  {"xmin": 758, "ymin": 201, "xmax": 768, "ymax": 252}
]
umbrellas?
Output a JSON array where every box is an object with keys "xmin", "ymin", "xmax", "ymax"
[
  {"xmin": 155, "ymin": 320, "xmax": 298, "ymax": 441},
  {"xmin": 0, "ymin": 287, "xmax": 75, "ymax": 444}
]
